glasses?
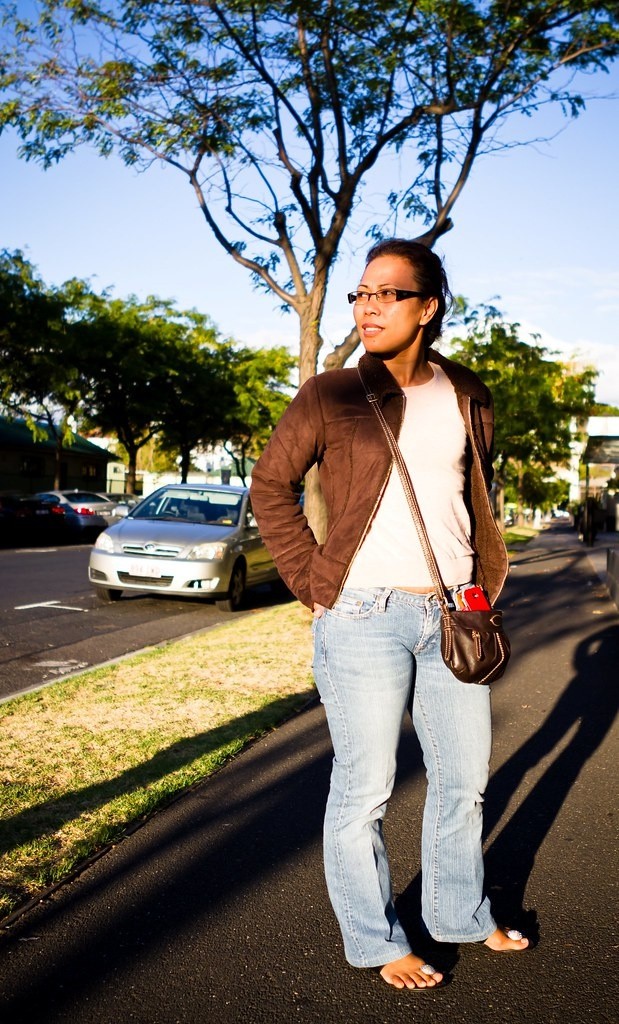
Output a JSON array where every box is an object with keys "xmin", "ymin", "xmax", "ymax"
[{"xmin": 347, "ymin": 288, "xmax": 422, "ymax": 305}]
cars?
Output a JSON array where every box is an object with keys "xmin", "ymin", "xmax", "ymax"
[
  {"xmin": 86, "ymin": 483, "xmax": 296, "ymax": 613},
  {"xmin": 40, "ymin": 491, "xmax": 118, "ymax": 539},
  {"xmin": 99, "ymin": 493, "xmax": 144, "ymax": 513},
  {"xmin": 0, "ymin": 493, "xmax": 81, "ymax": 550}
]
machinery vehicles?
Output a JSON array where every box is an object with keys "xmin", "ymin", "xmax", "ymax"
[{"xmin": 577, "ymin": 467, "xmax": 619, "ymax": 549}]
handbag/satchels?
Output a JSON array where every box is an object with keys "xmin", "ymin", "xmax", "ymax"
[{"xmin": 439, "ymin": 608, "xmax": 512, "ymax": 685}]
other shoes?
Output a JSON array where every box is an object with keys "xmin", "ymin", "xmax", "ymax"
[
  {"xmin": 474, "ymin": 924, "xmax": 535, "ymax": 954},
  {"xmin": 377, "ymin": 953, "xmax": 447, "ymax": 991}
]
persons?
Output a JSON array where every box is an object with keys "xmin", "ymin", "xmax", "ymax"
[{"xmin": 249, "ymin": 238, "xmax": 534, "ymax": 991}]
